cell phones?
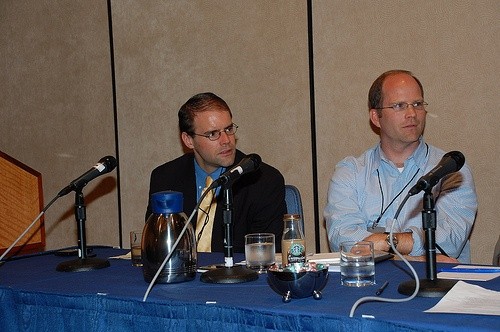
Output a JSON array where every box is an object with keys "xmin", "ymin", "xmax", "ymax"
[{"xmin": 56, "ymin": 247, "xmax": 93, "ymax": 255}]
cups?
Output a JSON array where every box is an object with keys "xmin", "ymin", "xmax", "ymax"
[
  {"xmin": 129, "ymin": 231, "xmax": 143, "ymax": 268},
  {"xmin": 244, "ymin": 233, "xmax": 275, "ymax": 273},
  {"xmin": 339, "ymin": 240, "xmax": 376, "ymax": 287}
]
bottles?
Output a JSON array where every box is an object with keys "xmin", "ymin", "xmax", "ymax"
[{"xmin": 281, "ymin": 213, "xmax": 307, "ymax": 267}]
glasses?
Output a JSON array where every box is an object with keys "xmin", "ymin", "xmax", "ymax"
[
  {"xmin": 186, "ymin": 122, "xmax": 238, "ymax": 142},
  {"xmin": 374, "ymin": 101, "xmax": 429, "ymax": 112}
]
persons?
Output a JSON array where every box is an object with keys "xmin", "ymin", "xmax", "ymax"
[
  {"xmin": 144, "ymin": 92, "xmax": 293, "ymax": 253},
  {"xmin": 323, "ymin": 70, "xmax": 479, "ymax": 265}
]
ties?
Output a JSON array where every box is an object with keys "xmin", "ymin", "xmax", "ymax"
[{"xmin": 196, "ymin": 177, "xmax": 217, "ymax": 252}]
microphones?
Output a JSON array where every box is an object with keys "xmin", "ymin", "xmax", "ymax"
[
  {"xmin": 209, "ymin": 153, "xmax": 263, "ymax": 188},
  {"xmin": 406, "ymin": 151, "xmax": 465, "ymax": 194},
  {"xmin": 58, "ymin": 155, "xmax": 118, "ymax": 196}
]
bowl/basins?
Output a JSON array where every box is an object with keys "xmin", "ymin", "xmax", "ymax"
[{"xmin": 267, "ymin": 263, "xmax": 329, "ymax": 303}]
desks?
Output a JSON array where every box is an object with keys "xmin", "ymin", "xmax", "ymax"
[{"xmin": 0, "ymin": 244, "xmax": 500, "ymax": 332}]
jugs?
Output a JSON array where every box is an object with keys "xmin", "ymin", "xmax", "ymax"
[{"xmin": 137, "ymin": 190, "xmax": 197, "ymax": 285}]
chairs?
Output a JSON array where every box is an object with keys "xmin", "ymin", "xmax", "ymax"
[
  {"xmin": 286, "ymin": 185, "xmax": 305, "ymax": 235},
  {"xmin": 0, "ymin": 150, "xmax": 46, "ymax": 257}
]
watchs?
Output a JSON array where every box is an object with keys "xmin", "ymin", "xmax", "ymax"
[{"xmin": 383, "ymin": 232, "xmax": 399, "ymax": 253}]
